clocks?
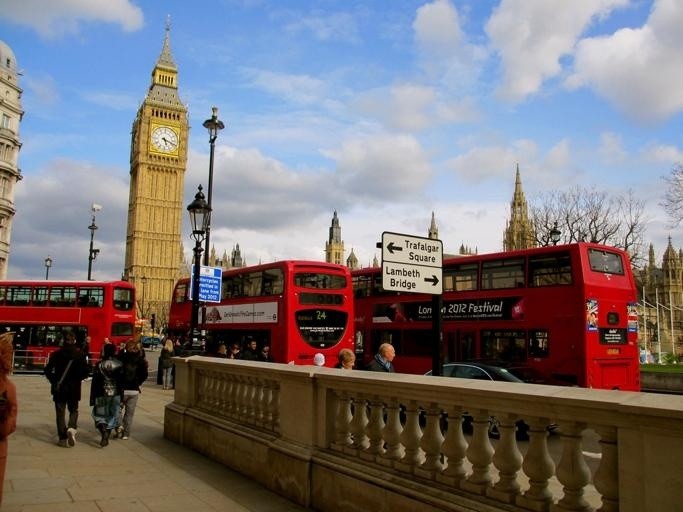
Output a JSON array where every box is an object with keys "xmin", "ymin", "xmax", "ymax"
[{"xmin": 147, "ymin": 118, "xmax": 183, "ymax": 160}]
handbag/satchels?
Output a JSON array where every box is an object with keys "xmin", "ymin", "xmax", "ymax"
[{"xmin": 51, "ymin": 381, "xmax": 58, "ymax": 394}]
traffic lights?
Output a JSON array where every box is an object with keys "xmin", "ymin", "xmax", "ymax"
[{"xmin": 151, "ymin": 313, "xmax": 156, "ymax": 329}]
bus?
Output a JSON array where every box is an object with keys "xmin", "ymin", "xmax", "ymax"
[
  {"xmin": 168, "ymin": 259, "xmax": 354, "ymax": 374},
  {"xmin": 0, "ymin": 278, "xmax": 137, "ymax": 377},
  {"xmin": 352, "ymin": 237, "xmax": 643, "ymax": 393}
]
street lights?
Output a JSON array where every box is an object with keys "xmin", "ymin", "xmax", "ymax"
[
  {"xmin": 550, "ymin": 222, "xmax": 560, "ymax": 246},
  {"xmin": 187, "ymin": 185, "xmax": 213, "ymax": 355},
  {"xmin": 196, "ymin": 107, "xmax": 224, "ymax": 349},
  {"xmin": 43, "ymin": 256, "xmax": 54, "ymax": 281},
  {"xmin": 85, "ymin": 203, "xmax": 104, "ymax": 282}
]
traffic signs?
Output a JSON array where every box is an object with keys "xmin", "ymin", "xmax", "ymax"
[
  {"xmin": 190, "ymin": 265, "xmax": 222, "ymax": 302},
  {"xmin": 382, "ymin": 232, "xmax": 442, "ymax": 294}
]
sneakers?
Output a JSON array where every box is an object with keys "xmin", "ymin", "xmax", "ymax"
[
  {"xmin": 114, "ymin": 425, "xmax": 128, "ymax": 440},
  {"xmin": 56, "ymin": 439, "xmax": 68, "ymax": 446},
  {"xmin": 66, "ymin": 428, "xmax": 77, "ymax": 447}
]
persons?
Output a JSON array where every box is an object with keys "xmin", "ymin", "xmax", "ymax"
[
  {"xmin": 89, "ymin": 340, "xmax": 149, "ymax": 447},
  {"xmin": 43, "ymin": 332, "xmax": 87, "ymax": 447},
  {"xmin": 158, "ymin": 336, "xmax": 398, "ymax": 391},
  {"xmin": 488, "ymin": 336, "xmax": 548, "ymax": 356}
]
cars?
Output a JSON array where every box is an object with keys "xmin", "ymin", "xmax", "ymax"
[
  {"xmin": 652, "ymin": 352, "xmax": 680, "ymax": 365},
  {"xmin": 395, "ymin": 359, "xmax": 556, "ymax": 442},
  {"xmin": 142, "ymin": 337, "xmax": 159, "ymax": 346}
]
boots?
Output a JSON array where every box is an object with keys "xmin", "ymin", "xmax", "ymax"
[{"xmin": 99, "ymin": 425, "xmax": 110, "ymax": 447}]
